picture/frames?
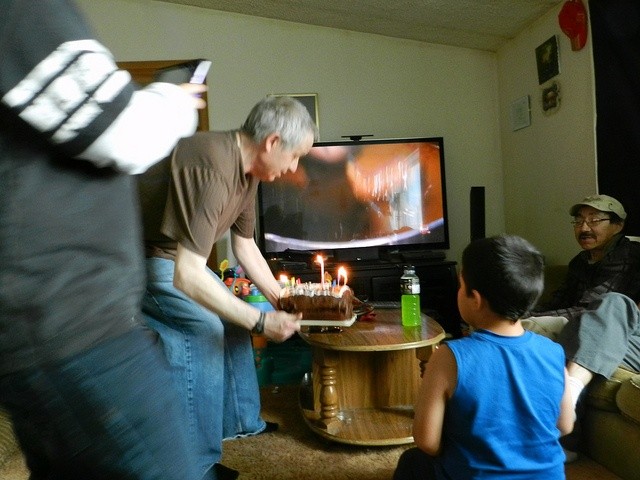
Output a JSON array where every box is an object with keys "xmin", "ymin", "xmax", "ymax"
[
  {"xmin": 269, "ymin": 89, "xmax": 322, "ymax": 148},
  {"xmin": 505, "ymin": 95, "xmax": 532, "ymax": 129},
  {"xmin": 530, "ymin": 35, "xmax": 565, "ymax": 84},
  {"xmin": 538, "ymin": 84, "xmax": 564, "ymax": 112}
]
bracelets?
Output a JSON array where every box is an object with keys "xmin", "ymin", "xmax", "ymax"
[{"xmin": 250, "ymin": 310, "xmax": 267, "ymax": 335}]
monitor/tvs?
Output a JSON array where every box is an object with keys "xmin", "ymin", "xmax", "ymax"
[{"xmin": 257, "ymin": 136, "xmax": 451, "ymax": 270}]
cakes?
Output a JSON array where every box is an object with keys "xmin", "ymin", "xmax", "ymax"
[{"xmin": 275, "ymin": 277, "xmax": 358, "ymax": 323}]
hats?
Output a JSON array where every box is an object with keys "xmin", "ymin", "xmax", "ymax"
[{"xmin": 570, "ymin": 195, "xmax": 627, "ymax": 220}]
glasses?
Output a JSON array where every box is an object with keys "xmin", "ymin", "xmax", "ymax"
[{"xmin": 571, "ymin": 216, "xmax": 619, "ymax": 225}]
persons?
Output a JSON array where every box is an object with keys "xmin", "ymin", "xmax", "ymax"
[
  {"xmin": 392, "ymin": 236, "xmax": 578, "ymax": 480},
  {"xmin": 563, "ymin": 194, "xmax": 640, "ymax": 407},
  {"xmin": 0, "ymin": 0, "xmax": 209, "ymax": 480},
  {"xmin": 140, "ymin": 95, "xmax": 317, "ymax": 478}
]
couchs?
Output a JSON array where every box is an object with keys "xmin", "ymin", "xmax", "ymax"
[{"xmin": 594, "ymin": 367, "xmax": 640, "ymax": 478}]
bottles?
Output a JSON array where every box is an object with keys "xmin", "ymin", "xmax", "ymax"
[{"xmin": 401, "ymin": 265, "xmax": 423, "ymax": 328}]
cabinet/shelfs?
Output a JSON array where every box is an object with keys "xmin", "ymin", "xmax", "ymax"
[{"xmin": 267, "ymin": 258, "xmax": 465, "ymax": 339}]
